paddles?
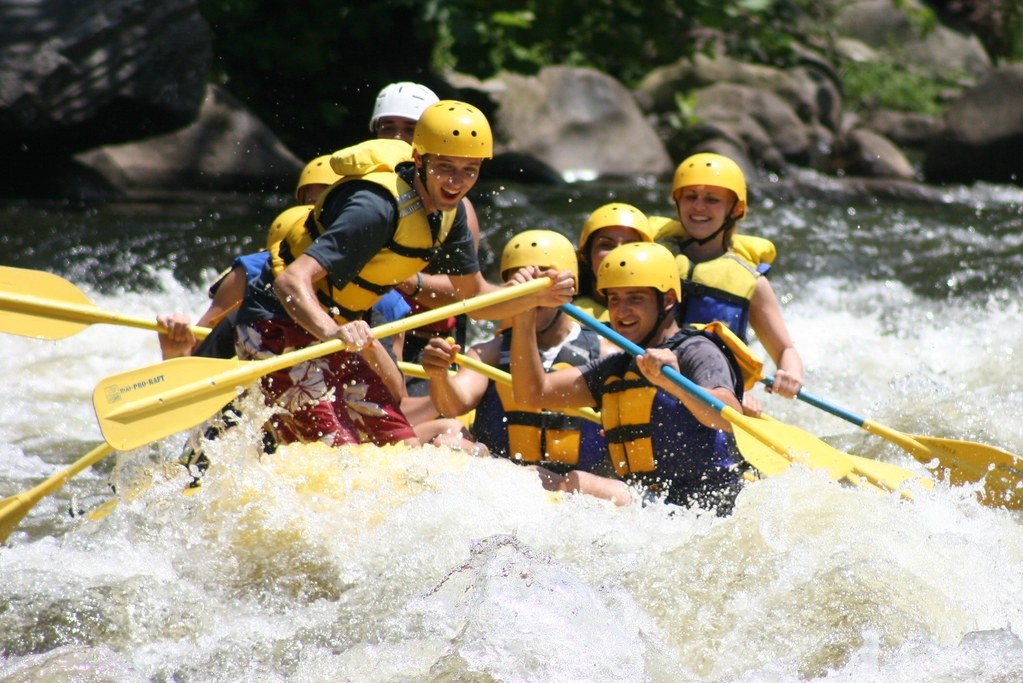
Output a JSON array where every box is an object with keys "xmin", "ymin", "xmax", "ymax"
[
  {"xmin": 1, "ymin": 264, "xmax": 456, "ymax": 382},
  {"xmin": 762, "ymin": 412, "xmax": 935, "ymax": 502},
  {"xmin": 445, "ymin": 335, "xmax": 603, "ymax": 425},
  {"xmin": 757, "ymin": 376, "xmax": 1023, "ymax": 510},
  {"xmin": 90, "ymin": 277, "xmax": 554, "ymax": 454},
  {"xmin": 0, "ymin": 441, "xmax": 114, "ymax": 546},
  {"xmin": 557, "ymin": 302, "xmax": 858, "ymax": 484}
]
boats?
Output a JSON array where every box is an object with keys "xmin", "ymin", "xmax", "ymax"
[{"xmin": 213, "ymin": 437, "xmax": 930, "ymax": 683}]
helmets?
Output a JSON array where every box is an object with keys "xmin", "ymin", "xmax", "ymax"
[
  {"xmin": 596, "ymin": 242, "xmax": 683, "ymax": 305},
  {"xmin": 268, "ymin": 204, "xmax": 318, "ymax": 246},
  {"xmin": 413, "ymin": 100, "xmax": 494, "ymax": 161},
  {"xmin": 499, "ymin": 229, "xmax": 578, "ymax": 296},
  {"xmin": 294, "ymin": 154, "xmax": 345, "ymax": 200},
  {"xmin": 673, "ymin": 153, "xmax": 747, "ymax": 220},
  {"xmin": 369, "ymin": 82, "xmax": 440, "ymax": 131},
  {"xmin": 578, "ymin": 203, "xmax": 654, "ymax": 262}
]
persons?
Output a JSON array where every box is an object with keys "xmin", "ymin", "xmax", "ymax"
[
  {"xmin": 646, "ymin": 153, "xmax": 803, "ymax": 400},
  {"xmin": 420, "ymin": 231, "xmax": 617, "ymax": 490},
  {"xmin": 572, "ymin": 204, "xmax": 762, "ymax": 416},
  {"xmin": 156, "ymin": 82, "xmax": 576, "ymax": 486},
  {"xmin": 505, "ymin": 243, "xmax": 759, "ymax": 517}
]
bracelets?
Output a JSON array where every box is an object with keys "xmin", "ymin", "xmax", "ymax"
[{"xmin": 410, "ymin": 272, "xmax": 422, "ymax": 297}]
